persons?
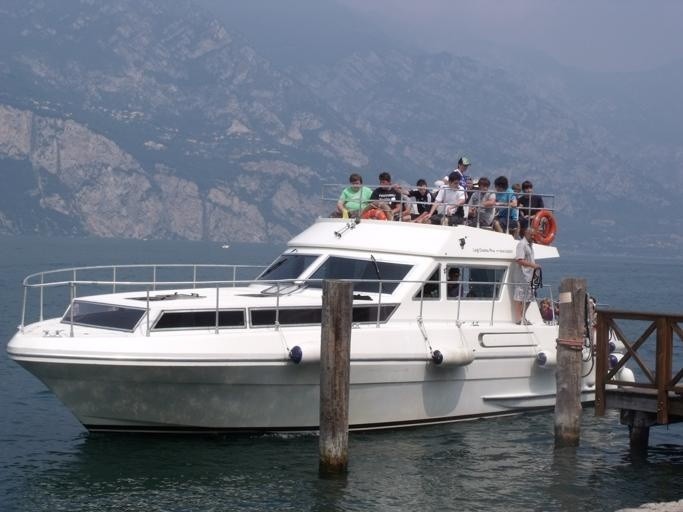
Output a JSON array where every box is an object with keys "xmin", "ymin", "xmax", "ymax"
[
  {"xmin": 330, "ymin": 156, "xmax": 543, "ymax": 241},
  {"xmin": 587, "ymin": 297, "xmax": 597, "ymax": 325},
  {"xmin": 447, "ymin": 267, "xmax": 462, "ymax": 298},
  {"xmin": 514, "ymin": 228, "xmax": 541, "ymax": 325},
  {"xmin": 539, "ymin": 298, "xmax": 553, "ymax": 322}
]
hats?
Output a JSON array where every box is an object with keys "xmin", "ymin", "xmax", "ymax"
[{"xmin": 457, "ymin": 156, "xmax": 472, "ymax": 166}]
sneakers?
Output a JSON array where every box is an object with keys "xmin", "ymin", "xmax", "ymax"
[{"xmin": 516, "ymin": 316, "xmax": 533, "ymax": 325}]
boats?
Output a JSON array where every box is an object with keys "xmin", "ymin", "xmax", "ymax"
[{"xmin": 7, "ymin": 183, "xmax": 638, "ymax": 439}]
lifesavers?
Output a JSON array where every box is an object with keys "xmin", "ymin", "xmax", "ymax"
[
  {"xmin": 361, "ymin": 202, "xmax": 394, "ymax": 221},
  {"xmin": 532, "ymin": 210, "xmax": 556, "ymax": 244}
]
